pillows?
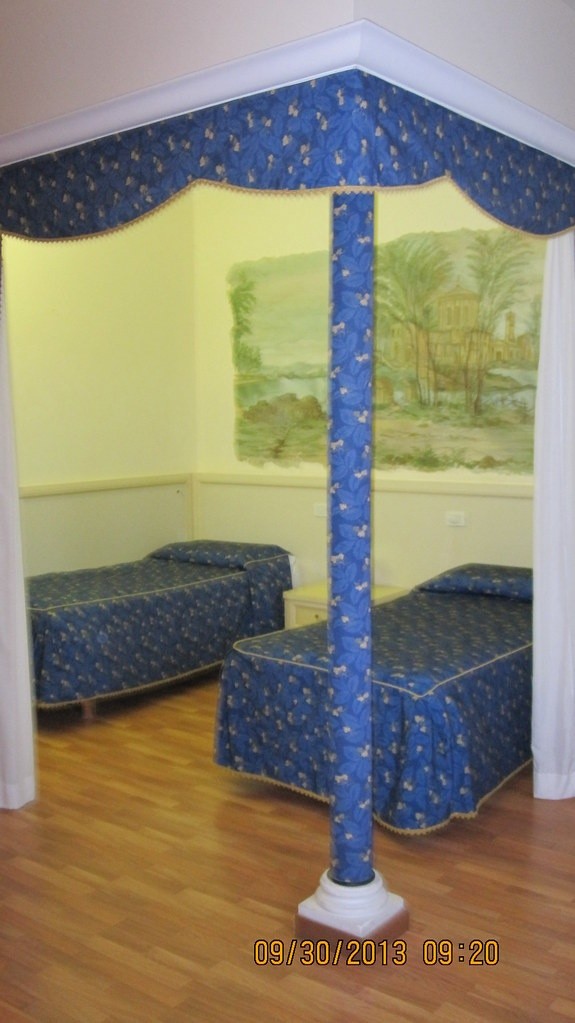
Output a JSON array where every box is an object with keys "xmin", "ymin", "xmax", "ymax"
[
  {"xmin": 410, "ymin": 562, "xmax": 532, "ymax": 601},
  {"xmin": 146, "ymin": 539, "xmax": 295, "ymax": 619}
]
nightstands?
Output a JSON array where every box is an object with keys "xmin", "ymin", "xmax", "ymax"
[{"xmin": 282, "ymin": 580, "xmax": 410, "ymax": 629}]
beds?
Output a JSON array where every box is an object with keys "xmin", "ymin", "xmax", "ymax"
[
  {"xmin": 25, "ymin": 554, "xmax": 284, "ymax": 720},
  {"xmin": 213, "ymin": 590, "xmax": 533, "ymax": 835}
]
